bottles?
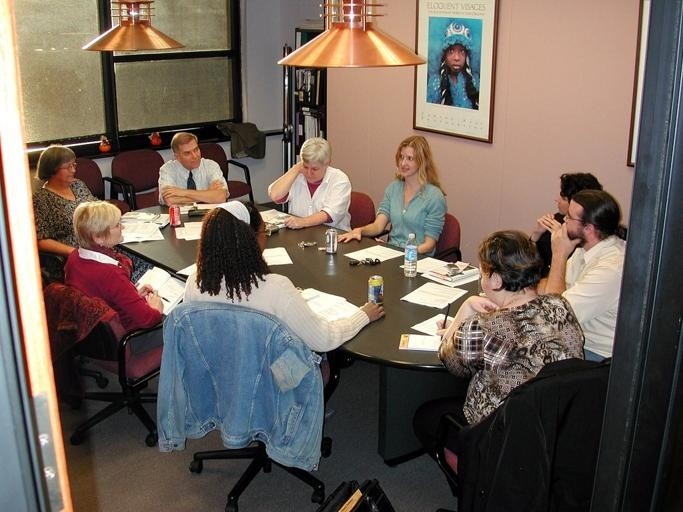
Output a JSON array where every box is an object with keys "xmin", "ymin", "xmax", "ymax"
[{"xmin": 403, "ymin": 233, "xmax": 417, "ymax": 278}]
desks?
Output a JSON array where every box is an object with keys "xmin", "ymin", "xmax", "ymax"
[{"xmin": 114, "ymin": 202, "xmax": 486, "ymax": 468}]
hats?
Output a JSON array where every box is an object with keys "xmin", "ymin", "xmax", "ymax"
[{"xmin": 442, "ymin": 19, "xmax": 471, "ymax": 57}]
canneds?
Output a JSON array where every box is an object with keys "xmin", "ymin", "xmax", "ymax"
[
  {"xmin": 169, "ymin": 204, "xmax": 181, "ymax": 227},
  {"xmin": 368, "ymin": 275, "xmax": 384, "ymax": 305},
  {"xmin": 325, "ymin": 229, "xmax": 337, "ymax": 255}
]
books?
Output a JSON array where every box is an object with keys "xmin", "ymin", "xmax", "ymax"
[
  {"xmin": 295, "ymin": 107, "xmax": 323, "ymax": 146},
  {"xmin": 121, "ymin": 211, "xmax": 170, "ymax": 229},
  {"xmin": 295, "ymin": 68, "xmax": 323, "ymax": 106},
  {"xmin": 429, "ymin": 260, "xmax": 479, "ymax": 282}
]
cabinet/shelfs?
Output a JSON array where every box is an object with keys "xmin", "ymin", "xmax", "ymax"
[{"xmin": 290, "ymin": 28, "xmax": 326, "ymax": 167}]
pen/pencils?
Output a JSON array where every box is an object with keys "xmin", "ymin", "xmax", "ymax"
[
  {"xmin": 148, "ymin": 289, "xmax": 170, "ymax": 304},
  {"xmin": 440, "ymin": 303, "xmax": 451, "ymax": 339}
]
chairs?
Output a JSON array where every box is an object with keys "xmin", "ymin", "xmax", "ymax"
[
  {"xmin": 435, "ymin": 357, "xmax": 611, "ymax": 512},
  {"xmin": 109, "ymin": 149, "xmax": 164, "ymax": 212},
  {"xmin": 371, "ymin": 214, "xmax": 461, "ymax": 263},
  {"xmin": 72, "ymin": 159, "xmax": 130, "ymax": 216},
  {"xmin": 43, "ymin": 282, "xmax": 164, "ymax": 448},
  {"xmin": 197, "ymin": 142, "xmax": 254, "ymax": 206},
  {"xmin": 161, "ymin": 300, "xmax": 333, "ymax": 512},
  {"xmin": 347, "ymin": 191, "xmax": 375, "ymax": 240}
]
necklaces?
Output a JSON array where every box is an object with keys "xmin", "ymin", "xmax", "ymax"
[{"xmin": 312, "ymin": 182, "xmax": 322, "ymax": 185}]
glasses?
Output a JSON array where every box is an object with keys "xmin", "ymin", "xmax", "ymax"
[
  {"xmin": 566, "ymin": 211, "xmax": 583, "ymax": 224},
  {"xmin": 254, "ymin": 228, "xmax": 271, "ymax": 236},
  {"xmin": 111, "ymin": 219, "xmax": 122, "ymax": 231},
  {"xmin": 57, "ymin": 162, "xmax": 78, "ymax": 170}
]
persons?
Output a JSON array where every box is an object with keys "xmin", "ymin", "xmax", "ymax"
[
  {"xmin": 530, "ymin": 172, "xmax": 604, "ymax": 278},
  {"xmin": 534, "ymin": 188, "xmax": 628, "ymax": 363},
  {"xmin": 158, "ymin": 132, "xmax": 231, "ymax": 207},
  {"xmin": 427, "ymin": 19, "xmax": 480, "ymax": 111},
  {"xmin": 183, "ymin": 199, "xmax": 386, "ymax": 370},
  {"xmin": 267, "ymin": 136, "xmax": 352, "ymax": 232},
  {"xmin": 32, "ymin": 145, "xmax": 155, "ymax": 291},
  {"xmin": 412, "ymin": 230, "xmax": 585, "ymax": 498},
  {"xmin": 336, "ymin": 136, "xmax": 448, "ymax": 257},
  {"xmin": 63, "ymin": 199, "xmax": 170, "ymax": 352}
]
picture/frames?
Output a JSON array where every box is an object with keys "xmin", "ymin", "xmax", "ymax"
[
  {"xmin": 411, "ymin": 0, "xmax": 500, "ymax": 145},
  {"xmin": 626, "ymin": 0, "xmax": 652, "ymax": 168}
]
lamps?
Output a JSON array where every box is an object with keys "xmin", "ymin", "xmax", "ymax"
[
  {"xmin": 80, "ymin": 1, "xmax": 186, "ymax": 52},
  {"xmin": 276, "ymin": 1, "xmax": 426, "ymax": 69}
]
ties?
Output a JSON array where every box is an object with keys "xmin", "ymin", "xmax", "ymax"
[{"xmin": 187, "ymin": 171, "xmax": 196, "ymax": 189}]
